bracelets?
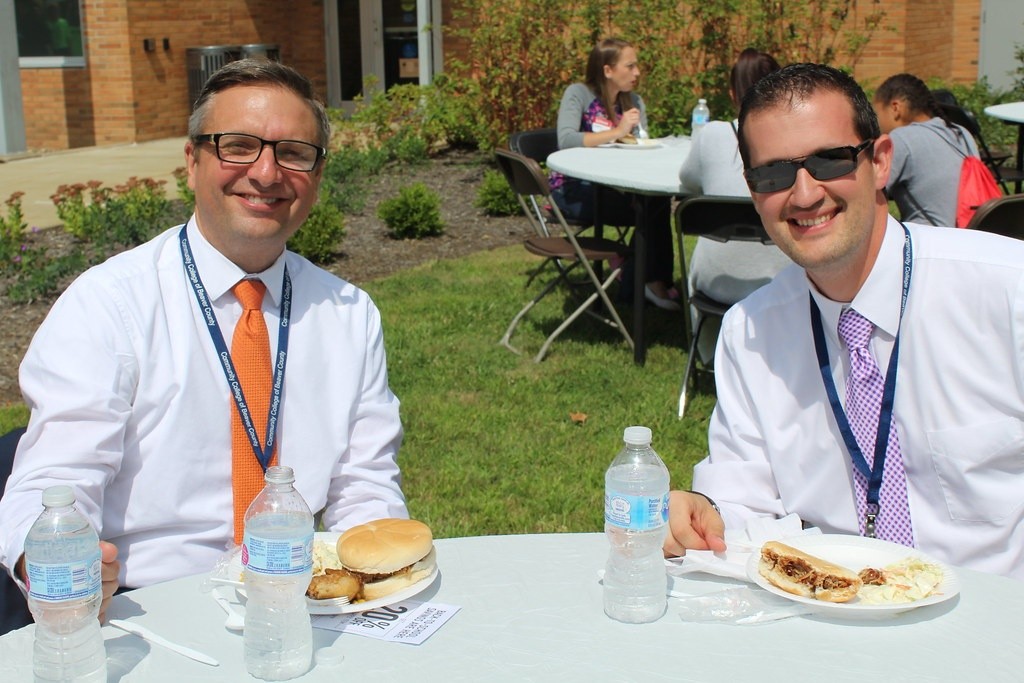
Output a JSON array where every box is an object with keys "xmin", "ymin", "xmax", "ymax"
[{"xmin": 682, "ymin": 490, "xmax": 722, "ymax": 515}]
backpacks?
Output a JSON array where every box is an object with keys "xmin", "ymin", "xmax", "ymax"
[{"xmin": 913, "ymin": 120, "xmax": 1003, "ymax": 229}]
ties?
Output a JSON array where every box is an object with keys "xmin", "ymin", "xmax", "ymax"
[
  {"xmin": 837, "ymin": 307, "xmax": 915, "ymax": 547},
  {"xmin": 229, "ymin": 279, "xmax": 277, "ymax": 544}
]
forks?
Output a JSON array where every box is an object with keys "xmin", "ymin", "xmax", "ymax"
[{"xmin": 211, "ymin": 578, "xmax": 349, "ymax": 607}]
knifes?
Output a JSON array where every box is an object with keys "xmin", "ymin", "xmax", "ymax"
[
  {"xmin": 109, "ymin": 619, "xmax": 219, "ymax": 666},
  {"xmin": 736, "ymin": 608, "xmax": 813, "ymax": 624}
]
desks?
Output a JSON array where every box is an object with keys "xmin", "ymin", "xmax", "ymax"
[
  {"xmin": 0, "ymin": 534, "xmax": 1024, "ymax": 683},
  {"xmin": 984, "ymin": 100, "xmax": 1024, "ymax": 193},
  {"xmin": 546, "ymin": 137, "xmax": 691, "ymax": 366}
]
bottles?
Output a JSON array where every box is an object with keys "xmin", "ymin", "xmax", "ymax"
[
  {"xmin": 604, "ymin": 426, "xmax": 670, "ymax": 623},
  {"xmin": 24, "ymin": 485, "xmax": 107, "ymax": 683},
  {"xmin": 691, "ymin": 99, "xmax": 710, "ymax": 141},
  {"xmin": 242, "ymin": 466, "xmax": 313, "ymax": 681}
]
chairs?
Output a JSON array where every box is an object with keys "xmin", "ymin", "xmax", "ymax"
[
  {"xmin": 932, "ymin": 88, "xmax": 1024, "ymax": 240},
  {"xmin": 491, "ymin": 129, "xmax": 779, "ymax": 423}
]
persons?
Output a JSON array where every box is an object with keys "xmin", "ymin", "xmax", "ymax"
[
  {"xmin": 678, "ymin": 47, "xmax": 792, "ymax": 369},
  {"xmin": 0, "ymin": 57, "xmax": 412, "ymax": 629},
  {"xmin": 867, "ymin": 74, "xmax": 988, "ymax": 230},
  {"xmin": 662, "ymin": 63, "xmax": 1024, "ymax": 581},
  {"xmin": 544, "ymin": 35, "xmax": 684, "ymax": 314}
]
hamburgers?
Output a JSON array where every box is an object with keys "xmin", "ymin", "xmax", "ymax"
[
  {"xmin": 614, "ymin": 133, "xmax": 638, "ymax": 144},
  {"xmin": 337, "ymin": 516, "xmax": 436, "ymax": 599},
  {"xmin": 757, "ymin": 541, "xmax": 863, "ymax": 604}
]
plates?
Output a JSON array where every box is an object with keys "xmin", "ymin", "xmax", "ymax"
[
  {"xmin": 615, "ymin": 139, "xmax": 659, "ymax": 149},
  {"xmin": 229, "ymin": 532, "xmax": 438, "ymax": 615},
  {"xmin": 745, "ymin": 534, "xmax": 961, "ymax": 621}
]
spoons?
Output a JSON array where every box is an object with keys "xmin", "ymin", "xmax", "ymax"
[
  {"xmin": 637, "ymin": 123, "xmax": 647, "ymax": 139},
  {"xmin": 213, "ymin": 588, "xmax": 245, "ymax": 630},
  {"xmin": 596, "ymin": 569, "xmax": 697, "ymax": 597}
]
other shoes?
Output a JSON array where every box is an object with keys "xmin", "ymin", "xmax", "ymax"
[
  {"xmin": 642, "ymin": 282, "xmax": 681, "ymax": 313},
  {"xmin": 694, "ymin": 359, "xmax": 714, "ymax": 374}
]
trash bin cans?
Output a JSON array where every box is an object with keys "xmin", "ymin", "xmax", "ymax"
[
  {"xmin": 241, "ymin": 44, "xmax": 280, "ymax": 62},
  {"xmin": 186, "ymin": 45, "xmax": 241, "ymax": 111}
]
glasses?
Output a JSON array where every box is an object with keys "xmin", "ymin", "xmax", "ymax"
[
  {"xmin": 190, "ymin": 132, "xmax": 327, "ymax": 172},
  {"xmin": 744, "ymin": 137, "xmax": 875, "ymax": 194}
]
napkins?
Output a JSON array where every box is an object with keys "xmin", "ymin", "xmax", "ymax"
[{"xmin": 664, "ymin": 502, "xmax": 822, "ymax": 600}]
